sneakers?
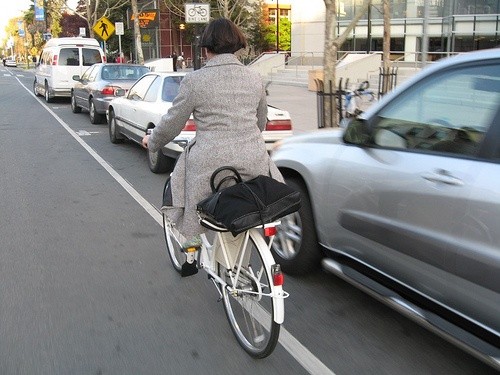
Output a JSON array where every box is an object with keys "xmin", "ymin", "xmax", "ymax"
[{"xmin": 181, "ymin": 236, "xmax": 202, "ymax": 251}]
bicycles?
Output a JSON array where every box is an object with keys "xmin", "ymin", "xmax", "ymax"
[{"xmin": 144, "ymin": 126, "xmax": 289, "ymax": 361}]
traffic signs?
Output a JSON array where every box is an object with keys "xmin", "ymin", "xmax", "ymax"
[
  {"xmin": 184, "ymin": 2, "xmax": 211, "ymax": 24},
  {"xmin": 115, "ymin": 22, "xmax": 125, "ymax": 36}
]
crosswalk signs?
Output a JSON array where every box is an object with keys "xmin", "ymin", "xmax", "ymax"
[{"xmin": 92, "ymin": 16, "xmax": 115, "ymax": 42}]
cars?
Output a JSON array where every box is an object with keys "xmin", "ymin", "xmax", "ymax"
[
  {"xmin": 259, "ymin": 48, "xmax": 500, "ymax": 371},
  {"xmin": 70, "ymin": 62, "xmax": 151, "ymax": 125},
  {"xmin": 106, "ymin": 71, "xmax": 292, "ymax": 174}
]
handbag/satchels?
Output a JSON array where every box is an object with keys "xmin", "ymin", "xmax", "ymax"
[{"xmin": 197, "ymin": 165, "xmax": 303, "ymax": 237}]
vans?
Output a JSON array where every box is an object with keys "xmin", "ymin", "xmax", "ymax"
[{"xmin": 32, "ymin": 36, "xmax": 108, "ymax": 103}]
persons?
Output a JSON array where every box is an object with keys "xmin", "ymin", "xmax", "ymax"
[
  {"xmin": 143, "ymin": 19, "xmax": 287, "ymax": 277},
  {"xmin": 113, "ymin": 58, "xmax": 117, "ymax": 62},
  {"xmin": 168, "ymin": 51, "xmax": 177, "ymax": 71},
  {"xmin": 176, "ymin": 52, "xmax": 185, "ymax": 69},
  {"xmin": 117, "ymin": 54, "xmax": 124, "ymax": 62}
]
